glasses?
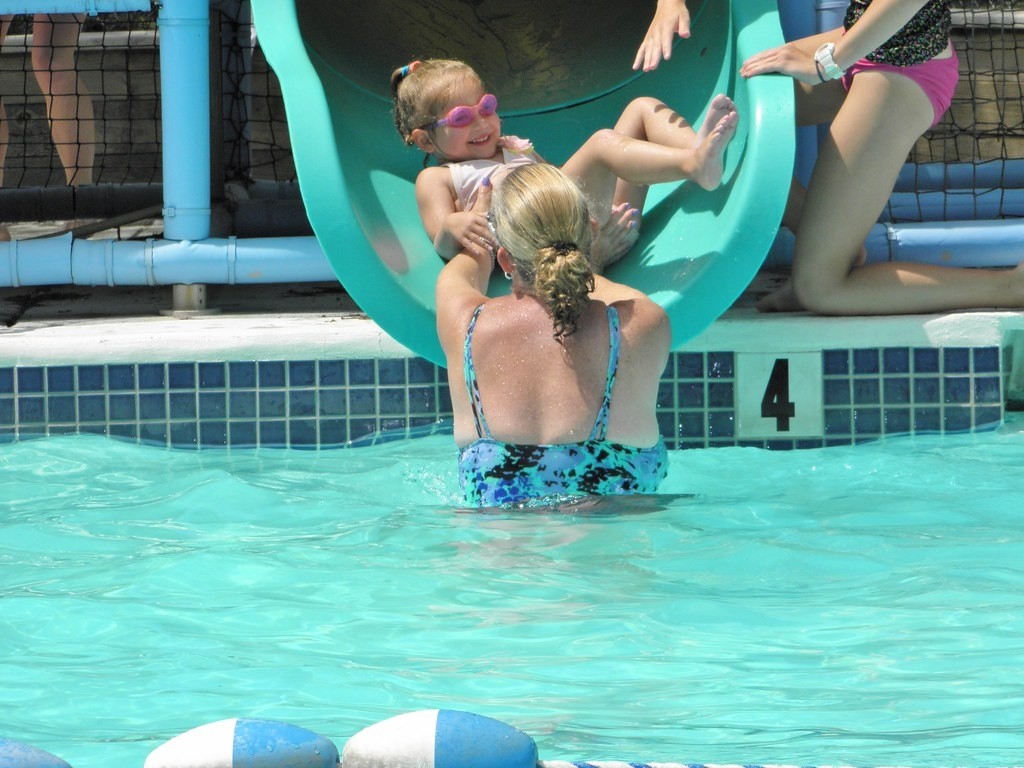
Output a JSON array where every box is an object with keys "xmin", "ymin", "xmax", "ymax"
[{"xmin": 421, "ymin": 94, "xmax": 498, "ymax": 132}]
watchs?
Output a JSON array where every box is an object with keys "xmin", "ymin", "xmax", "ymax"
[{"xmin": 814, "ymin": 42, "xmax": 846, "ymax": 81}]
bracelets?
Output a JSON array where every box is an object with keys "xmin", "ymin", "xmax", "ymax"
[
  {"xmin": 816, "ymin": 61, "xmax": 827, "ymax": 83},
  {"xmin": 479, "ymin": 237, "xmax": 496, "ymax": 271}
]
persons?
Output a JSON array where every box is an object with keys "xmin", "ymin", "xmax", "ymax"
[
  {"xmin": 0, "ymin": 14, "xmax": 95, "ymax": 242},
  {"xmin": 633, "ymin": 1, "xmax": 1024, "ymax": 316},
  {"xmin": 390, "ymin": 59, "xmax": 737, "ymax": 273},
  {"xmin": 434, "ymin": 162, "xmax": 672, "ymax": 505}
]
